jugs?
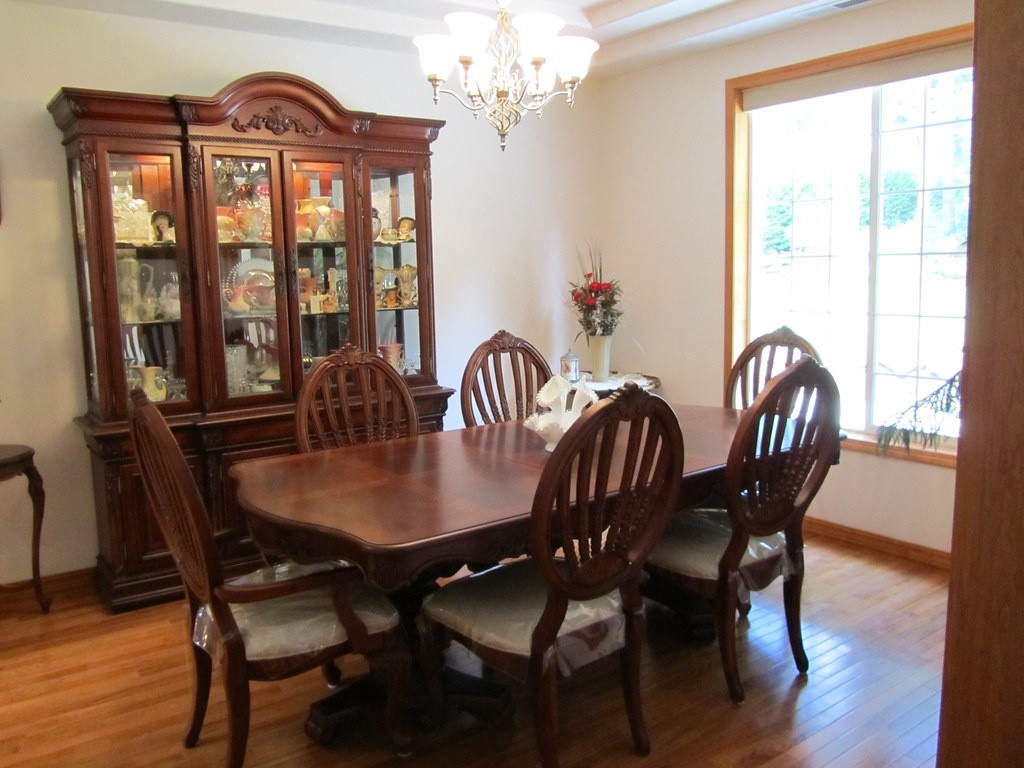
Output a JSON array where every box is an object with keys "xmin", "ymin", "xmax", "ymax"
[
  {"xmin": 223, "ymin": 285, "xmax": 250, "ymax": 313},
  {"xmin": 133, "ymin": 366, "xmax": 168, "ymax": 402}
]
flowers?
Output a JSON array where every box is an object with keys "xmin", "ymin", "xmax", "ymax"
[{"xmin": 568, "ymin": 240, "xmax": 624, "ymax": 348}]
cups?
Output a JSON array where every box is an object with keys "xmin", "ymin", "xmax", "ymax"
[{"xmin": 561, "ymin": 349, "xmax": 580, "ymax": 382}]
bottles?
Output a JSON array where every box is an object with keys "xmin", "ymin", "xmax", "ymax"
[
  {"xmin": 378, "ymin": 343, "xmax": 405, "ymax": 375},
  {"xmin": 309, "ymin": 196, "xmax": 331, "ymax": 233},
  {"xmin": 372, "ymin": 208, "xmax": 381, "ymax": 240},
  {"xmin": 295, "ymin": 199, "xmax": 314, "ymax": 228}
]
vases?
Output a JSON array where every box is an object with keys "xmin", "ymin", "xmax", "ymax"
[
  {"xmin": 588, "ymin": 335, "xmax": 612, "ymax": 381},
  {"xmin": 379, "ymin": 344, "xmax": 405, "ymax": 376}
]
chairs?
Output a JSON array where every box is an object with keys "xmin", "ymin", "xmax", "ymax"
[
  {"xmin": 295, "ymin": 343, "xmax": 419, "ymax": 454},
  {"xmin": 638, "ymin": 354, "xmax": 840, "ymax": 704},
  {"xmin": 691, "ymin": 324, "xmax": 823, "ymax": 614},
  {"xmin": 461, "ymin": 328, "xmax": 556, "ymax": 427},
  {"xmin": 128, "ymin": 389, "xmax": 414, "ymax": 768},
  {"xmin": 416, "ymin": 381, "xmax": 686, "ymax": 768}
]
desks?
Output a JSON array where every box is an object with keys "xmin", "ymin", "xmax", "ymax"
[
  {"xmin": 0, "ymin": 442, "xmax": 52, "ymax": 613},
  {"xmin": 558, "ymin": 373, "xmax": 661, "ymax": 400},
  {"xmin": 227, "ymin": 404, "xmax": 845, "ymax": 742}
]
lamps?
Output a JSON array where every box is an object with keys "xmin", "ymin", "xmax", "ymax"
[{"xmin": 412, "ymin": 0, "xmax": 600, "ymax": 151}]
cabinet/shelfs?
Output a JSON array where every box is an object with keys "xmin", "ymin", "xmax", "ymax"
[{"xmin": 46, "ymin": 72, "xmax": 457, "ymax": 615}]
